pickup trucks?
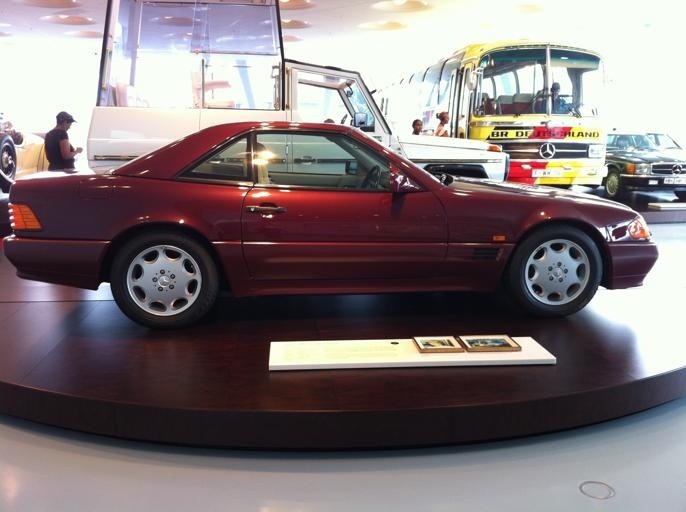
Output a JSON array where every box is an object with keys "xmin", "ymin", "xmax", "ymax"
[{"xmin": 89, "ymin": 54, "xmax": 516, "ymax": 187}]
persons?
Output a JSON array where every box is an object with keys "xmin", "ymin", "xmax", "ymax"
[
  {"xmin": 542, "ymin": 82, "xmax": 566, "ymax": 113},
  {"xmin": 435, "ymin": 111, "xmax": 451, "ymax": 136},
  {"xmin": 42, "ymin": 110, "xmax": 82, "ymax": 171},
  {"xmin": 411, "ymin": 119, "xmax": 423, "ymax": 135}
]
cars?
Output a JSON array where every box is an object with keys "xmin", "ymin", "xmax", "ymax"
[
  {"xmin": 602, "ymin": 126, "xmax": 686, "ymax": 201},
  {"xmin": 0, "ymin": 110, "xmax": 55, "ymax": 192}
]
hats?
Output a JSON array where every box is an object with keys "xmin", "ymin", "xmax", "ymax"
[{"xmin": 54, "ymin": 110, "xmax": 78, "ymax": 125}]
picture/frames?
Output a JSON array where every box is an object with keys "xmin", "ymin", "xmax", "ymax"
[
  {"xmin": 455, "ymin": 334, "xmax": 520, "ymax": 352},
  {"xmin": 412, "ymin": 335, "xmax": 464, "ymax": 353}
]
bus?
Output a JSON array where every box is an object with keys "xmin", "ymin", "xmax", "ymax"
[{"xmin": 369, "ymin": 38, "xmax": 610, "ymax": 188}]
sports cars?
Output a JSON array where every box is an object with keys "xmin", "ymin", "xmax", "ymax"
[{"xmin": 6, "ymin": 122, "xmax": 667, "ymax": 323}]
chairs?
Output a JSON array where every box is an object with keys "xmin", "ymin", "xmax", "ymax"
[
  {"xmin": 243, "ymin": 142, "xmax": 270, "ymax": 184},
  {"xmin": 479, "ymin": 92, "xmax": 534, "ymax": 114}
]
